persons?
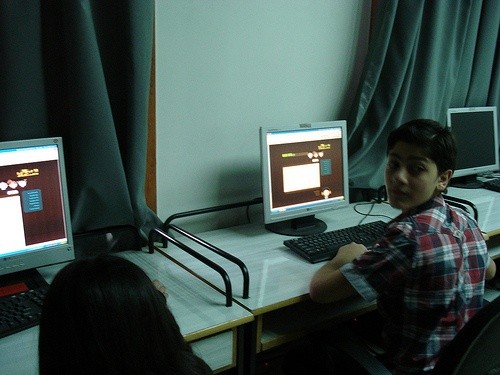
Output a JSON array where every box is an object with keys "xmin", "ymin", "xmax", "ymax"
[
  {"xmin": 309, "ymin": 118, "xmax": 496, "ymax": 375},
  {"xmin": 37, "ymin": 253, "xmax": 214, "ymax": 375}
]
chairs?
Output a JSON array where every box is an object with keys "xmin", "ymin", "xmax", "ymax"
[{"xmin": 315, "ymin": 285, "xmax": 500, "ymax": 375}]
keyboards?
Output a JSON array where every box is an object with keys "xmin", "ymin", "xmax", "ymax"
[
  {"xmin": 283, "ymin": 221, "xmax": 388, "ymax": 263},
  {"xmin": 483, "ymin": 179, "xmax": 500, "ymax": 191},
  {"xmin": 0, "ymin": 285, "xmax": 51, "ymax": 338}
]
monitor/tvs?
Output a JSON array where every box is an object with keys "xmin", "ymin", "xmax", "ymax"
[
  {"xmin": 260, "ymin": 119, "xmax": 350, "ymax": 236},
  {"xmin": 447, "ymin": 107, "xmax": 499, "ymax": 188},
  {"xmin": 0, "ymin": 137, "xmax": 76, "ymax": 296}
]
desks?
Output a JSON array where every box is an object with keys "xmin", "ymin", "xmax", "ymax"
[{"xmin": 0, "ymin": 183, "xmax": 500, "ymax": 375}]
